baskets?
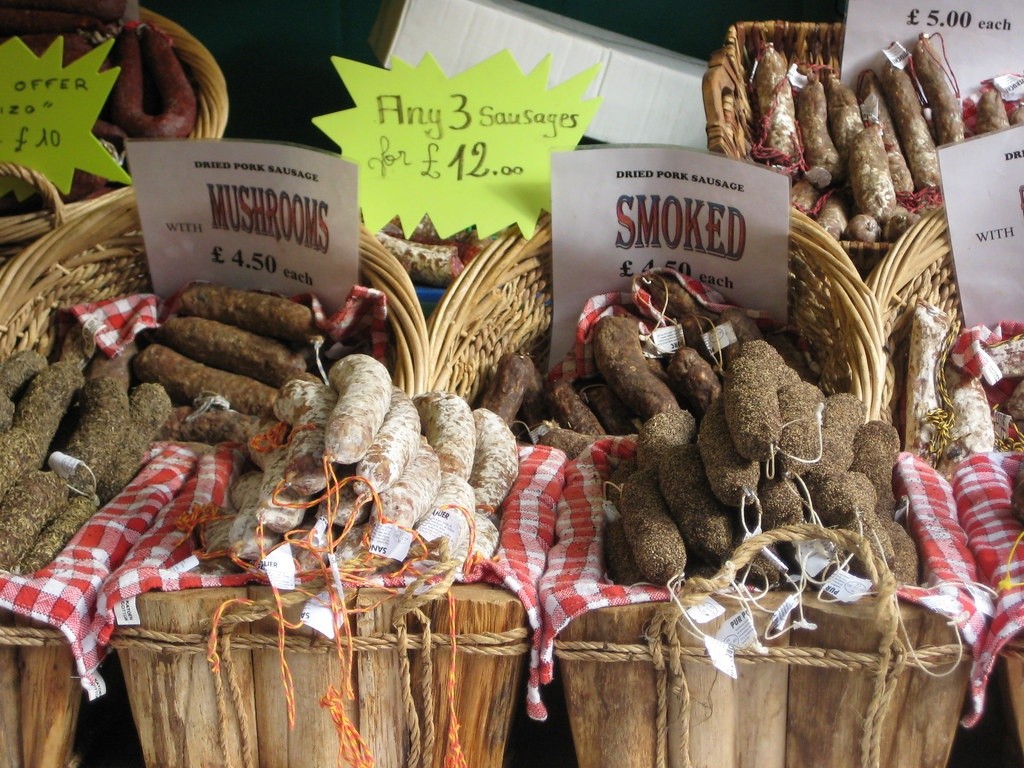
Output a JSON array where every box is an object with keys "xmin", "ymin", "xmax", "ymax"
[{"xmin": 1, "ymin": 1, "xmax": 1024, "ymax": 427}]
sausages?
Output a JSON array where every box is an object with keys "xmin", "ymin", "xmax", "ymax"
[
  {"xmin": 0, "ymin": 270, "xmax": 1024, "ymax": 594},
  {"xmin": 0, "ymin": 0, "xmax": 197, "ymax": 198},
  {"xmin": 756, "ymin": 34, "xmax": 1024, "ymax": 241}
]
